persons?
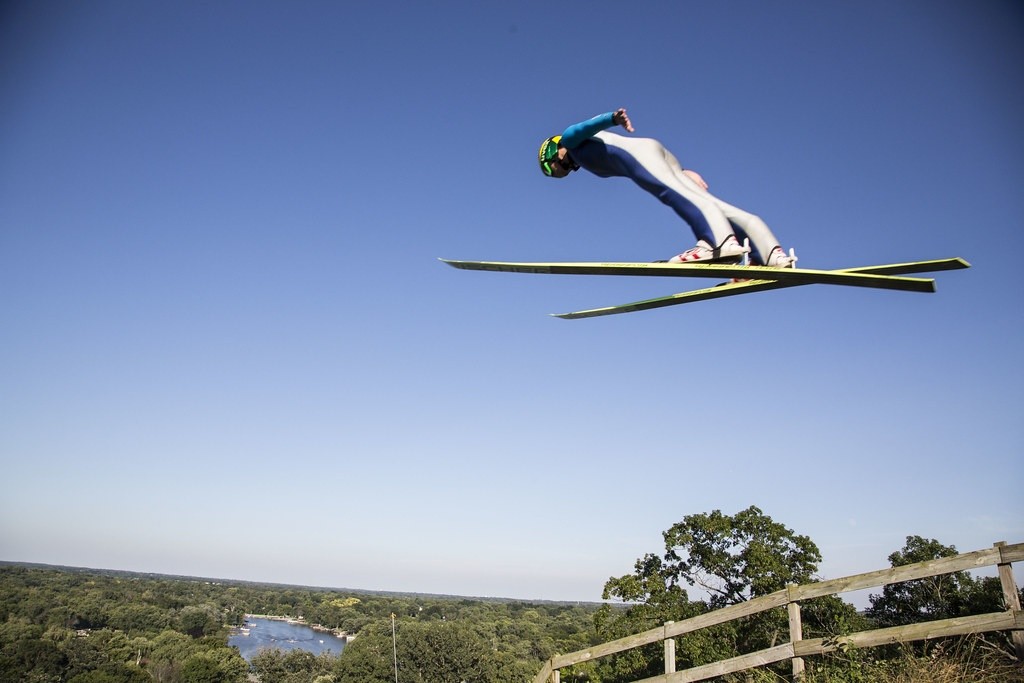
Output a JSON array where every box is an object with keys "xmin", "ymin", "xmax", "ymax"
[{"xmin": 540, "ymin": 108, "xmax": 801, "ymax": 285}]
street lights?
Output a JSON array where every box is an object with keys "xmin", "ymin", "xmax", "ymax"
[{"xmin": 391, "ymin": 612, "xmax": 399, "ymax": 683}]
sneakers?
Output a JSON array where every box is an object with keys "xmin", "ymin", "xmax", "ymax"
[
  {"xmin": 715, "ymin": 245, "xmax": 791, "ymax": 287},
  {"xmin": 667, "ymin": 234, "xmax": 744, "ymax": 263}
]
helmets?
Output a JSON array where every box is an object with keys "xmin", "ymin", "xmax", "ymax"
[{"xmin": 538, "ymin": 135, "xmax": 572, "ymax": 178}]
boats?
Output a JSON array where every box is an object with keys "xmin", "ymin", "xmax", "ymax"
[{"xmin": 239, "ymin": 622, "xmax": 257, "ymax": 636}]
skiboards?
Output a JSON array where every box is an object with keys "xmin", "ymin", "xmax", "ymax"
[{"xmin": 432, "ymin": 246, "xmax": 983, "ymax": 325}]
